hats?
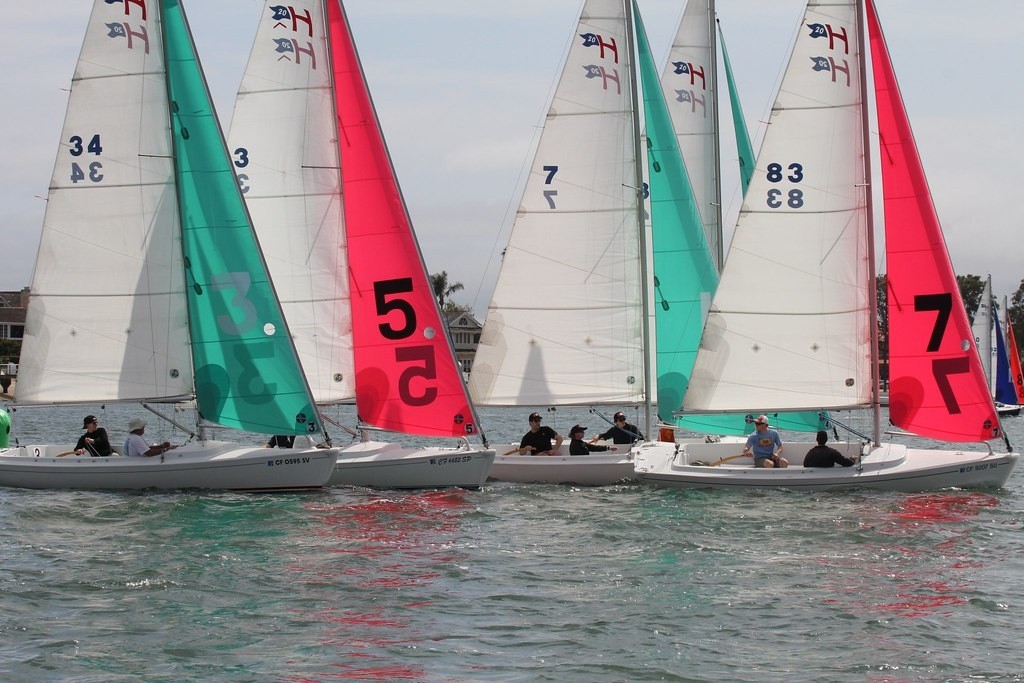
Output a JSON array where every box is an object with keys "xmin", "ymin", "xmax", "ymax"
[
  {"xmin": 754, "ymin": 415, "xmax": 768, "ymax": 424},
  {"xmin": 571, "ymin": 425, "xmax": 587, "ymax": 434},
  {"xmin": 816, "ymin": 431, "xmax": 828, "ymax": 440},
  {"xmin": 613, "ymin": 411, "xmax": 627, "ymax": 422},
  {"xmin": 128, "ymin": 418, "xmax": 147, "ymax": 433},
  {"xmin": 529, "ymin": 412, "xmax": 542, "ymax": 422},
  {"xmin": 82, "ymin": 415, "xmax": 97, "ymax": 429}
]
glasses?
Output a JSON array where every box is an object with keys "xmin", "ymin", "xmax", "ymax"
[
  {"xmin": 90, "ymin": 421, "xmax": 98, "ymax": 425},
  {"xmin": 755, "ymin": 422, "xmax": 764, "ymax": 426},
  {"xmin": 618, "ymin": 418, "xmax": 626, "ymax": 422},
  {"xmin": 577, "ymin": 431, "xmax": 584, "ymax": 433},
  {"xmin": 531, "ymin": 419, "xmax": 541, "ymax": 423}
]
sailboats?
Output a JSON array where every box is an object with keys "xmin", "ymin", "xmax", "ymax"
[
  {"xmin": 138, "ymin": 0, "xmax": 494, "ymax": 493},
  {"xmin": 509, "ymin": 1, "xmax": 754, "ymax": 449},
  {"xmin": 0, "ymin": 1, "xmax": 362, "ymax": 495},
  {"xmin": 412, "ymin": 0, "xmax": 759, "ymax": 489},
  {"xmin": 595, "ymin": 0, "xmax": 1024, "ymax": 495}
]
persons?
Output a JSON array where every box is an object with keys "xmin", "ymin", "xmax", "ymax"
[
  {"xmin": 122, "ymin": 418, "xmax": 179, "ymax": 457},
  {"xmin": 262, "ymin": 435, "xmax": 296, "ymax": 448},
  {"xmin": 520, "ymin": 412, "xmax": 563, "ymax": 456},
  {"xmin": 568, "ymin": 425, "xmax": 618, "ymax": 455},
  {"xmin": 593, "ymin": 412, "xmax": 639, "ymax": 444},
  {"xmin": 75, "ymin": 415, "xmax": 119, "ymax": 457},
  {"xmin": 803, "ymin": 431, "xmax": 858, "ymax": 467},
  {"xmin": 743, "ymin": 415, "xmax": 789, "ymax": 468}
]
